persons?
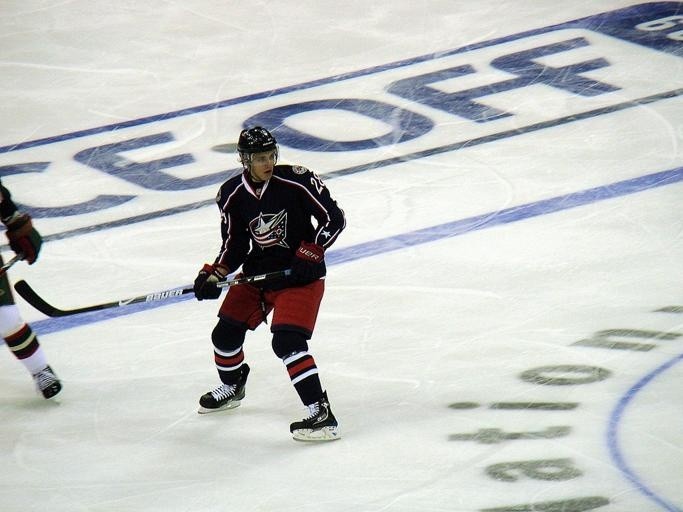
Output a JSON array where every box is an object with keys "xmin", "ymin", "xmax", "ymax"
[
  {"xmin": 0, "ymin": 177, "xmax": 63, "ymax": 401},
  {"xmin": 188, "ymin": 123, "xmax": 348, "ymax": 436}
]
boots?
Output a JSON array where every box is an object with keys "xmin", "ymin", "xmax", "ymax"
[
  {"xmin": 290, "ymin": 390, "xmax": 338, "ymax": 434},
  {"xmin": 199, "ymin": 364, "xmax": 250, "ymax": 408}
]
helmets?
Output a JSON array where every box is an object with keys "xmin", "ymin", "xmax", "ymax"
[{"xmin": 237, "ymin": 127, "xmax": 277, "ymax": 154}]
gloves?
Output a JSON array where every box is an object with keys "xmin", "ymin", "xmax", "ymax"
[
  {"xmin": 193, "ymin": 264, "xmax": 228, "ymax": 301},
  {"xmin": 290, "ymin": 240, "xmax": 326, "ymax": 279},
  {"xmin": 6, "ymin": 212, "xmax": 42, "ymax": 264}
]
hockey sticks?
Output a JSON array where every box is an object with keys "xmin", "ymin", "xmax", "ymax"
[{"xmin": 14, "ymin": 269, "xmax": 292, "ymax": 318}]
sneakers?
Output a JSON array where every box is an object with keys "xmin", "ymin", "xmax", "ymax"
[{"xmin": 33, "ymin": 365, "xmax": 61, "ymax": 399}]
